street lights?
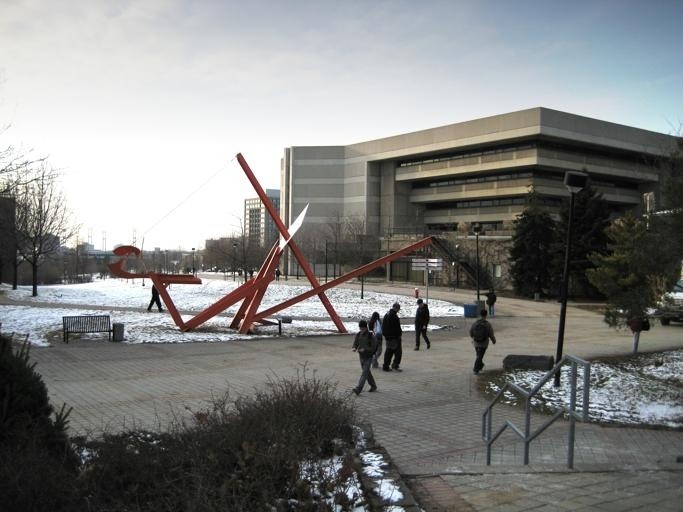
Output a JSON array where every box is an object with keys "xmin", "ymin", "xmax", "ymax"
[
  {"xmin": 553, "ymin": 172, "xmax": 588, "ymax": 387},
  {"xmin": 473, "ymin": 226, "xmax": 483, "ymax": 300},
  {"xmin": 192, "ymin": 247, "xmax": 196, "ymax": 272},
  {"xmin": 233, "ymin": 243, "xmax": 238, "ymax": 281}
]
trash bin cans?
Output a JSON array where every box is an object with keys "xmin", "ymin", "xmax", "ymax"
[
  {"xmin": 113, "ymin": 323, "xmax": 124, "ymax": 342},
  {"xmin": 464, "ymin": 300, "xmax": 485, "ymax": 317}
]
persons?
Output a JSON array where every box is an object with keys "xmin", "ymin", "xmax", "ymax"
[
  {"xmin": 350, "ymin": 320, "xmax": 381, "ymax": 396},
  {"xmin": 413, "ymin": 298, "xmax": 431, "ymax": 351},
  {"xmin": 368, "ymin": 312, "xmax": 383, "ymax": 368},
  {"xmin": 383, "ymin": 303, "xmax": 404, "ymax": 372},
  {"xmin": 147, "ymin": 283, "xmax": 165, "ymax": 312},
  {"xmin": 275, "ymin": 269, "xmax": 281, "ymax": 282},
  {"xmin": 469, "ymin": 310, "xmax": 496, "ymax": 376},
  {"xmin": 480, "ymin": 285, "xmax": 497, "ymax": 316}
]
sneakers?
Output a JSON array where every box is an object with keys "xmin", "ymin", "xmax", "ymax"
[
  {"xmin": 383, "ymin": 367, "xmax": 392, "ymax": 371},
  {"xmin": 369, "ymin": 385, "xmax": 377, "ymax": 392},
  {"xmin": 392, "ymin": 365, "xmax": 402, "ymax": 372},
  {"xmin": 352, "ymin": 389, "xmax": 360, "ymax": 396},
  {"xmin": 426, "ymin": 342, "xmax": 429, "ymax": 349}
]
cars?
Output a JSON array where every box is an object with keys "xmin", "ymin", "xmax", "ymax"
[{"xmin": 657, "ymin": 279, "xmax": 683, "ymax": 326}]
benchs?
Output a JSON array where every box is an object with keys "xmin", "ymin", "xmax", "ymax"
[{"xmin": 62, "ymin": 315, "xmax": 113, "ymax": 344}]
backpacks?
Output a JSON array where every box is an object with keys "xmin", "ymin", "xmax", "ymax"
[{"xmin": 356, "ymin": 331, "xmax": 378, "ymax": 355}]
